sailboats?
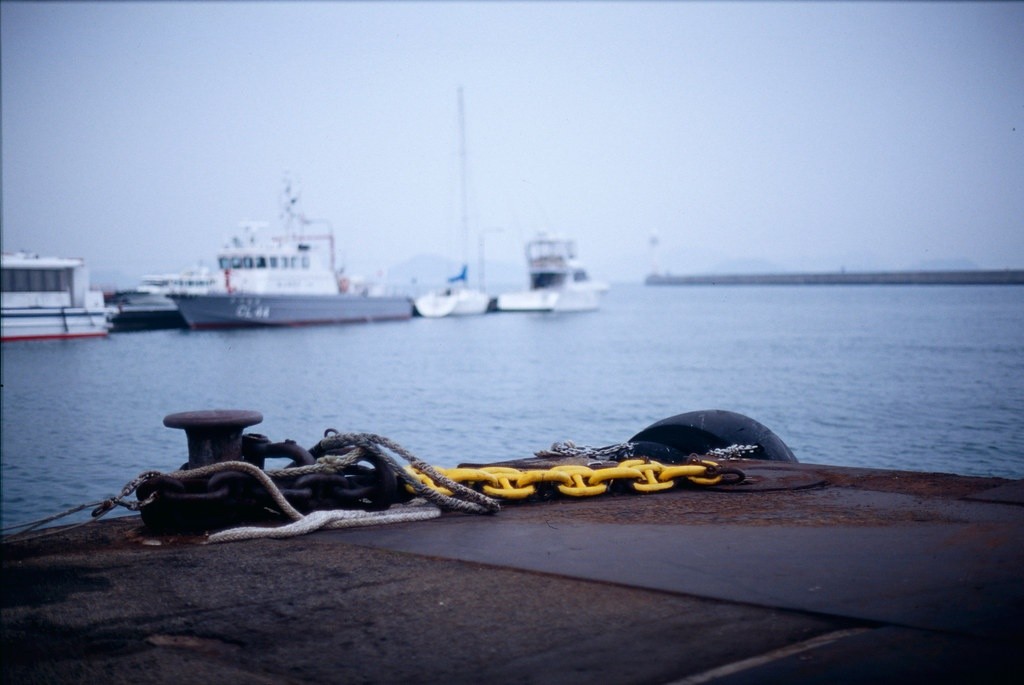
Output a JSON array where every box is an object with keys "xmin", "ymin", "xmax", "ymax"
[{"xmin": 415, "ymin": 74, "xmax": 493, "ymax": 319}]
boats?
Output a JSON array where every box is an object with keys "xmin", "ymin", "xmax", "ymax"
[
  {"xmin": 106, "ymin": 270, "xmax": 214, "ymax": 331},
  {"xmin": 0, "ymin": 253, "xmax": 109, "ymax": 341},
  {"xmin": 496, "ymin": 232, "xmax": 609, "ymax": 311},
  {"xmin": 164, "ymin": 193, "xmax": 415, "ymax": 333}
]
